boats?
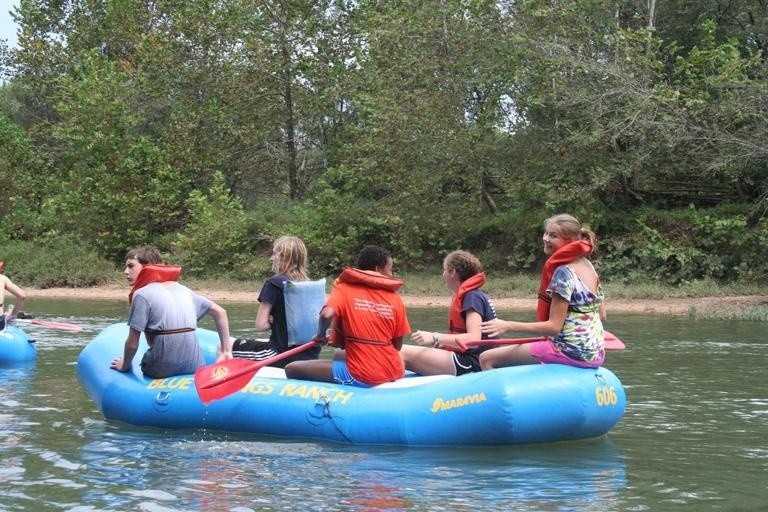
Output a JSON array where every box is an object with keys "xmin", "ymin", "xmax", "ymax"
[
  {"xmin": 75, "ymin": 318, "xmax": 626, "ymax": 444},
  {"xmin": 0, "ymin": 326, "xmax": 38, "ymax": 365}
]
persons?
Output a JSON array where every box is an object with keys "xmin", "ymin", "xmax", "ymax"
[
  {"xmin": 109, "ymin": 245, "xmax": 234, "ymax": 378},
  {"xmin": 284, "ymin": 246, "xmax": 412, "ymax": 388},
  {"xmin": 0, "ymin": 262, "xmax": 26, "ymax": 331},
  {"xmin": 217, "ymin": 236, "xmax": 321, "ymax": 370},
  {"xmin": 398, "ymin": 249, "xmax": 502, "ymax": 376},
  {"xmin": 478, "ymin": 213, "xmax": 607, "ymax": 372}
]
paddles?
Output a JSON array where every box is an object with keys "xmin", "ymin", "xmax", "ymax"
[
  {"xmin": 455, "ymin": 330, "xmax": 626, "ymax": 352},
  {"xmin": 11, "ymin": 319, "xmax": 83, "ymax": 332},
  {"xmin": 194, "ymin": 336, "xmax": 329, "ymax": 404}
]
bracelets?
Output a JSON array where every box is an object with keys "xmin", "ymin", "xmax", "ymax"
[{"xmin": 430, "ymin": 331, "xmax": 442, "ymax": 349}]
